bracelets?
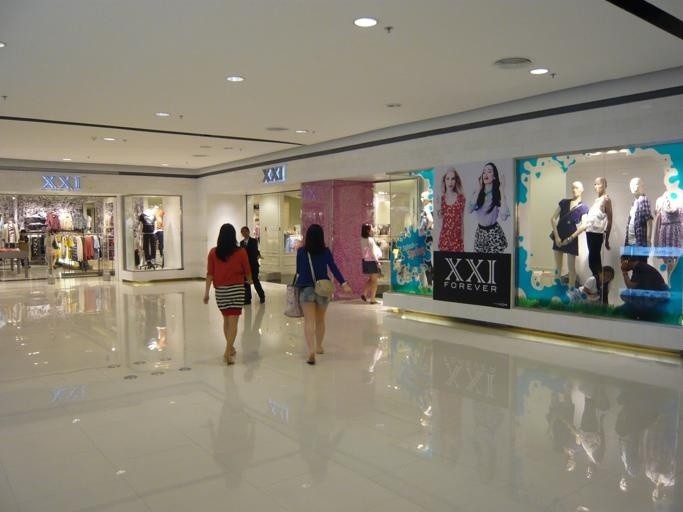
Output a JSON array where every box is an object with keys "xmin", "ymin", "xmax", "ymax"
[
  {"xmin": 566, "ymin": 237, "xmax": 570, "ymax": 244},
  {"xmin": 570, "ymin": 235, "xmax": 573, "ymax": 242},
  {"xmin": 622, "ymin": 271, "xmax": 628, "ymax": 277}
]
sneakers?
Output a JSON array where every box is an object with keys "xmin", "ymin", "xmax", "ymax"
[
  {"xmin": 260, "ymin": 294, "xmax": 265, "ymax": 303},
  {"xmin": 243, "ymin": 298, "xmax": 252, "ymax": 304},
  {"xmin": 361, "ymin": 294, "xmax": 367, "ymax": 302},
  {"xmin": 307, "ymin": 357, "xmax": 316, "ymax": 365},
  {"xmin": 224, "ymin": 347, "xmax": 237, "ymax": 365},
  {"xmin": 371, "ymin": 302, "xmax": 378, "ymax": 304}
]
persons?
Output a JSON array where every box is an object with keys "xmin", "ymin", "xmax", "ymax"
[
  {"xmin": 548, "ymin": 181, "xmax": 589, "ymax": 288},
  {"xmin": 207, "ymin": 362, "xmax": 257, "ymax": 490},
  {"xmin": 43, "ymin": 228, "xmax": 59, "ymax": 270},
  {"xmin": 568, "ymin": 266, "xmax": 614, "ymax": 303},
  {"xmin": 239, "ymin": 302, "xmax": 266, "ymax": 380},
  {"xmin": 2, "ymin": 221, "xmax": 19, "ymax": 248},
  {"xmin": 614, "ymin": 254, "xmax": 670, "ymax": 320},
  {"xmin": 239, "ymin": 226, "xmax": 264, "ymax": 303},
  {"xmin": 134, "ymin": 209, "xmax": 157, "ymax": 269},
  {"xmin": 584, "ymin": 177, "xmax": 612, "ymax": 313},
  {"xmin": 415, "ymin": 191, "xmax": 433, "ymax": 286},
  {"xmin": 467, "ymin": 162, "xmax": 509, "ymax": 253},
  {"xmin": 620, "ymin": 177, "xmax": 653, "ymax": 264},
  {"xmin": 294, "ymin": 224, "xmax": 351, "ymax": 363},
  {"xmin": 651, "ymin": 168, "xmax": 682, "ymax": 276},
  {"xmin": 18, "ymin": 230, "xmax": 31, "ymax": 268},
  {"xmin": 151, "ymin": 205, "xmax": 164, "ymax": 268},
  {"xmin": 539, "ymin": 360, "xmax": 681, "ymax": 505},
  {"xmin": 202, "ymin": 223, "xmax": 252, "ymax": 364},
  {"xmin": 360, "ymin": 223, "xmax": 381, "ymax": 304},
  {"xmin": 434, "ymin": 167, "xmax": 465, "ymax": 252},
  {"xmin": 289, "ymin": 362, "xmax": 346, "ymax": 482}
]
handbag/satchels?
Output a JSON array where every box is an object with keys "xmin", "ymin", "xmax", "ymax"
[
  {"xmin": 315, "ymin": 279, "xmax": 336, "ymax": 297},
  {"xmin": 284, "ymin": 273, "xmax": 305, "ymax": 318},
  {"xmin": 362, "ymin": 236, "xmax": 383, "ymax": 262}
]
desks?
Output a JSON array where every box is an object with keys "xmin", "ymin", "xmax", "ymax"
[{"xmin": 0, "ymin": 249, "xmax": 29, "ymax": 278}]
